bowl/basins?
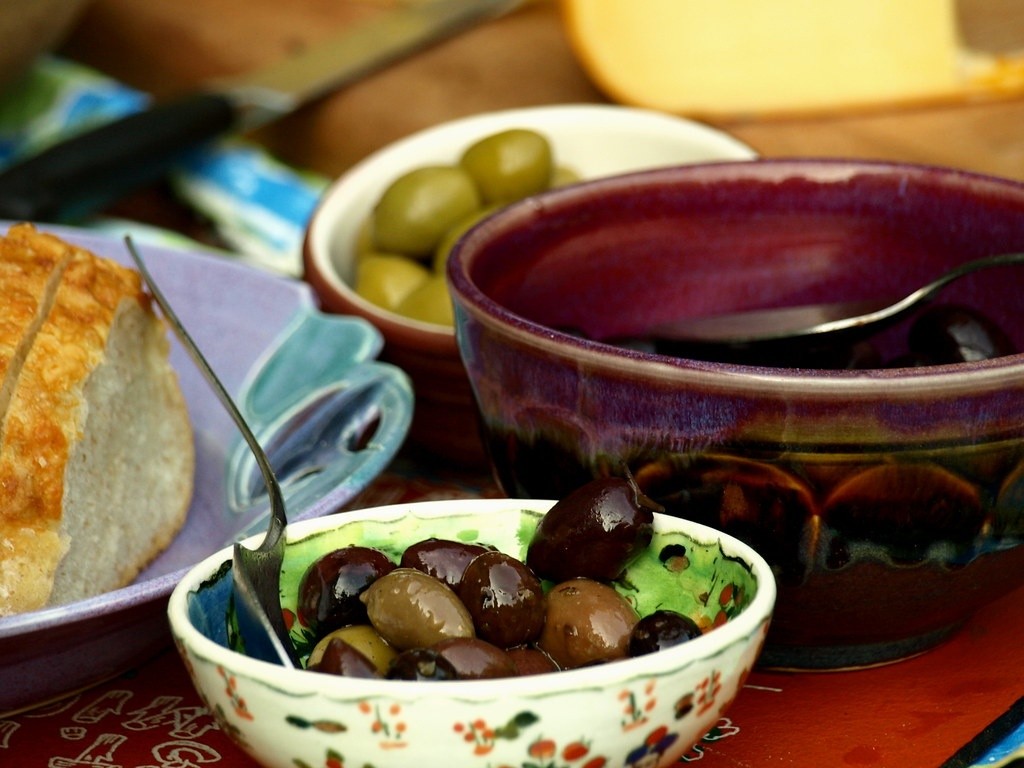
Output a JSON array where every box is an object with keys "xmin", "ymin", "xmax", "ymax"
[
  {"xmin": 0, "ymin": 214, "xmax": 414, "ymax": 643},
  {"xmin": 446, "ymin": 157, "xmax": 1024, "ymax": 673},
  {"xmin": 167, "ymin": 498, "xmax": 777, "ymax": 768},
  {"xmin": 306, "ymin": 101, "xmax": 760, "ymax": 407}
]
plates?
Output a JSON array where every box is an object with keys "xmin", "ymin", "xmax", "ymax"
[{"xmin": 0, "ymin": 51, "xmax": 335, "ymax": 279}]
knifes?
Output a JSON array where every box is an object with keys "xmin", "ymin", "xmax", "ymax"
[{"xmin": 0, "ymin": 0, "xmax": 550, "ymax": 234}]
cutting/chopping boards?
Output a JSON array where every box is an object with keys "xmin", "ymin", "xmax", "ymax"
[{"xmin": 0, "ymin": 0, "xmax": 1024, "ymax": 182}]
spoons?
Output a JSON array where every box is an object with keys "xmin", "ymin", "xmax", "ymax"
[
  {"xmin": 611, "ymin": 252, "xmax": 1024, "ymax": 355},
  {"xmin": 124, "ymin": 231, "xmax": 302, "ymax": 671}
]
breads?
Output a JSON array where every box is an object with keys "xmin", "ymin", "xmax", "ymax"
[{"xmin": 0, "ymin": 220, "xmax": 194, "ymax": 618}]
